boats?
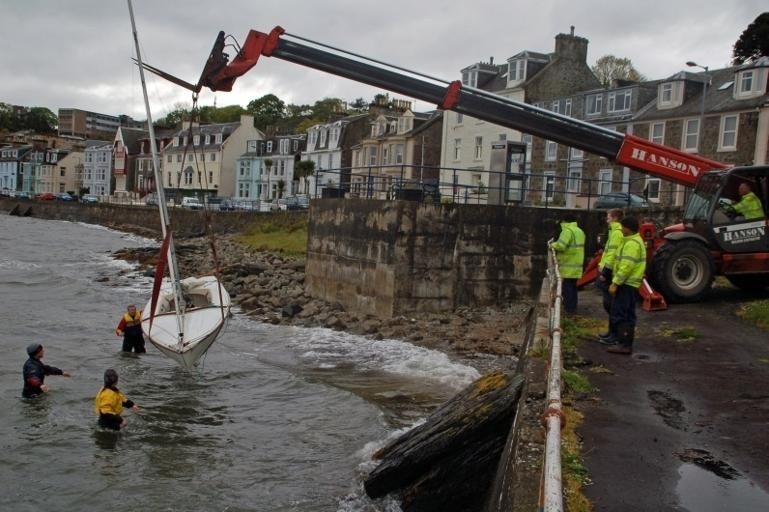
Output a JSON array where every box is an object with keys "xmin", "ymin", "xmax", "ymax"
[{"xmin": 139, "ymin": 274, "xmax": 232, "ymax": 372}]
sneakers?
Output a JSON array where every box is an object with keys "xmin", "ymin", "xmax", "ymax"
[{"xmin": 599, "ymin": 332, "xmax": 632, "ymax": 355}]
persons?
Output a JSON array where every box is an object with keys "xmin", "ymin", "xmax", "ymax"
[
  {"xmin": 546, "ymin": 211, "xmax": 588, "ymax": 316},
  {"xmin": 606, "ymin": 217, "xmax": 651, "ymax": 357},
  {"xmin": 19, "ymin": 341, "xmax": 72, "ymax": 397},
  {"xmin": 114, "ymin": 303, "xmax": 147, "ymax": 357},
  {"xmin": 718, "ymin": 182, "xmax": 766, "ymax": 225},
  {"xmin": 597, "ymin": 207, "xmax": 625, "ymax": 346},
  {"xmin": 93, "ymin": 366, "xmax": 140, "ymax": 430}
]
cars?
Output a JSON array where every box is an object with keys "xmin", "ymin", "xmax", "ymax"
[
  {"xmin": 219, "ymin": 199, "xmax": 243, "ymax": 210},
  {"xmin": 82, "ymin": 194, "xmax": 98, "ymax": 203},
  {"xmin": 1, "ymin": 189, "xmax": 73, "ymax": 202},
  {"xmin": 269, "ymin": 194, "xmax": 311, "ymax": 214},
  {"xmin": 593, "ymin": 191, "xmax": 653, "ymax": 209}
]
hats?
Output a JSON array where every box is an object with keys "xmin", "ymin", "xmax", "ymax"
[
  {"xmin": 27, "ymin": 343, "xmax": 42, "ymax": 357},
  {"xmin": 621, "ymin": 216, "xmax": 638, "ymax": 233}
]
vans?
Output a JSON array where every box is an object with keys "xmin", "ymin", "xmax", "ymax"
[{"xmin": 182, "ymin": 197, "xmax": 203, "ymax": 209}]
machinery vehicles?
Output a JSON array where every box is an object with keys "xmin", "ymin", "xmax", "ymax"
[{"xmin": 133, "ymin": 26, "xmax": 768, "ymax": 314}]
